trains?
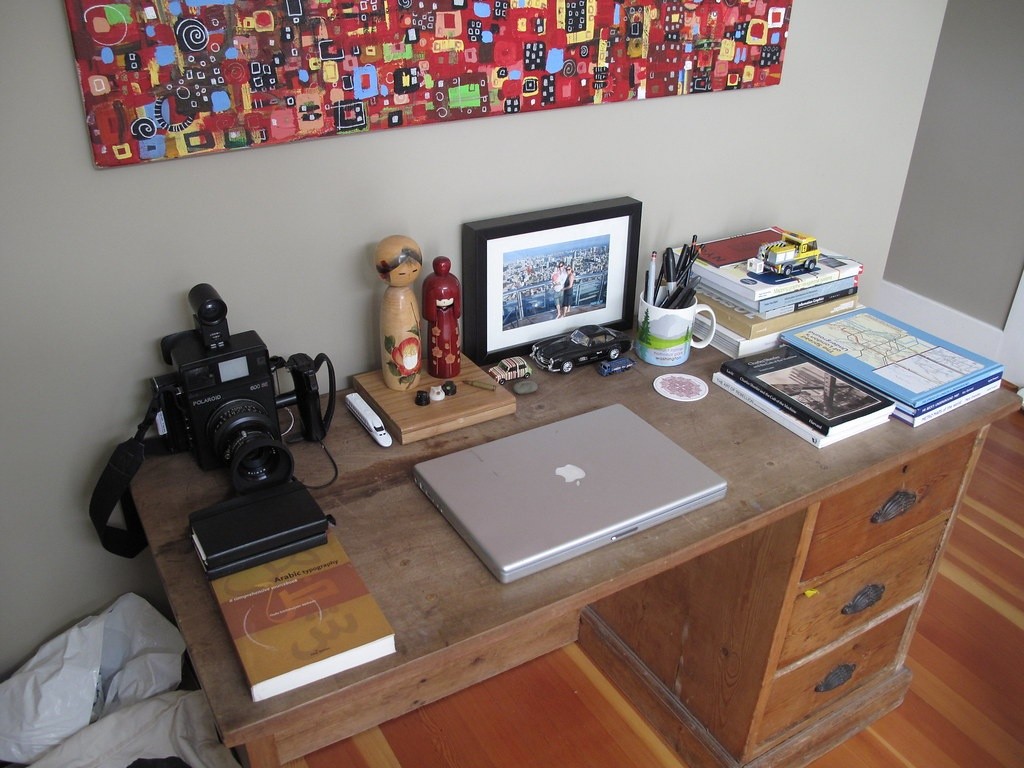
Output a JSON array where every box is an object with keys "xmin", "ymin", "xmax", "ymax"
[{"xmin": 344, "ymin": 392, "xmax": 393, "ymax": 448}]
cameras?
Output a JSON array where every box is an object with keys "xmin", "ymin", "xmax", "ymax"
[{"xmin": 150, "ymin": 283, "xmax": 325, "ymax": 494}]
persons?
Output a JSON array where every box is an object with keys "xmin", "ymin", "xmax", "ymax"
[
  {"xmin": 552, "ymin": 261, "xmax": 567, "ymax": 319},
  {"xmin": 562, "ymin": 265, "xmax": 574, "ymax": 316},
  {"xmin": 551, "ymin": 267, "xmax": 561, "ymax": 283}
]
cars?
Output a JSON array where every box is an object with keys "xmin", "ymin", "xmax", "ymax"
[
  {"xmin": 488, "ymin": 356, "xmax": 532, "ymax": 385},
  {"xmin": 529, "ymin": 324, "xmax": 632, "ymax": 374}
]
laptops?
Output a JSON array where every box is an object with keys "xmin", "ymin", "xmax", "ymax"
[{"xmin": 412, "ymin": 404, "xmax": 728, "ymax": 584}]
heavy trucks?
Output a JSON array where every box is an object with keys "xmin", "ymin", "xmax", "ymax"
[{"xmin": 755, "ymin": 231, "xmax": 821, "ymax": 277}]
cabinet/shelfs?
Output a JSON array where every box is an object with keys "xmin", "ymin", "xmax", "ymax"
[{"xmin": 576, "ymin": 429, "xmax": 986, "ymax": 768}]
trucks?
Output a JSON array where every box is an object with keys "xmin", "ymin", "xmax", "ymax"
[{"xmin": 597, "ymin": 356, "xmax": 636, "ymax": 376}]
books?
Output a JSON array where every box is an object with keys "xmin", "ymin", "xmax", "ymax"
[
  {"xmin": 664, "ymin": 225, "xmax": 864, "ymax": 360},
  {"xmin": 207, "ymin": 524, "xmax": 397, "ymax": 703},
  {"xmin": 711, "ymin": 345, "xmax": 897, "ymax": 448},
  {"xmin": 778, "ymin": 306, "xmax": 1005, "ymax": 428}
]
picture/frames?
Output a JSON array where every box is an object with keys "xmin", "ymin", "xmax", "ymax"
[{"xmin": 461, "ymin": 195, "xmax": 644, "ymax": 368}]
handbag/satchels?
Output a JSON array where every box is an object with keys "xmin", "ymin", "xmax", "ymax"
[{"xmin": 0, "ymin": 592, "xmax": 186, "ymax": 764}]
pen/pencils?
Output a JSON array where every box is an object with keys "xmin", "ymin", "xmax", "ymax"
[{"xmin": 643, "ymin": 235, "xmax": 705, "ymax": 309}]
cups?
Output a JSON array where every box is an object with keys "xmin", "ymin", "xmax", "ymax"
[{"xmin": 635, "ymin": 286, "xmax": 716, "ymax": 366}]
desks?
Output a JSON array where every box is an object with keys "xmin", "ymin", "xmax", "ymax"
[{"xmin": 126, "ymin": 285, "xmax": 1023, "ymax": 768}]
